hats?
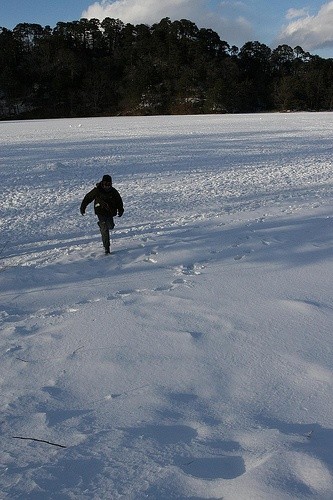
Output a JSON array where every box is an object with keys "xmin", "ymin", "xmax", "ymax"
[{"xmin": 102, "ymin": 175, "xmax": 112, "ymax": 182}]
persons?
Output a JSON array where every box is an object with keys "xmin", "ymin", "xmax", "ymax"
[{"xmin": 79, "ymin": 174, "xmax": 124, "ymax": 255}]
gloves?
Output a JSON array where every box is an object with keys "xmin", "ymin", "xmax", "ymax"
[
  {"xmin": 80, "ymin": 207, "xmax": 85, "ymax": 216},
  {"xmin": 118, "ymin": 212, "xmax": 123, "ymax": 218}
]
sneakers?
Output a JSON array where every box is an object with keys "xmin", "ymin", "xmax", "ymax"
[
  {"xmin": 104, "ymin": 246, "xmax": 110, "ymax": 254},
  {"xmin": 97, "ymin": 222, "xmax": 101, "ymax": 228}
]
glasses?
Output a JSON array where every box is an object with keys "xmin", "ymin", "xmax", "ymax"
[{"xmin": 104, "ymin": 183, "xmax": 112, "ymax": 186}]
